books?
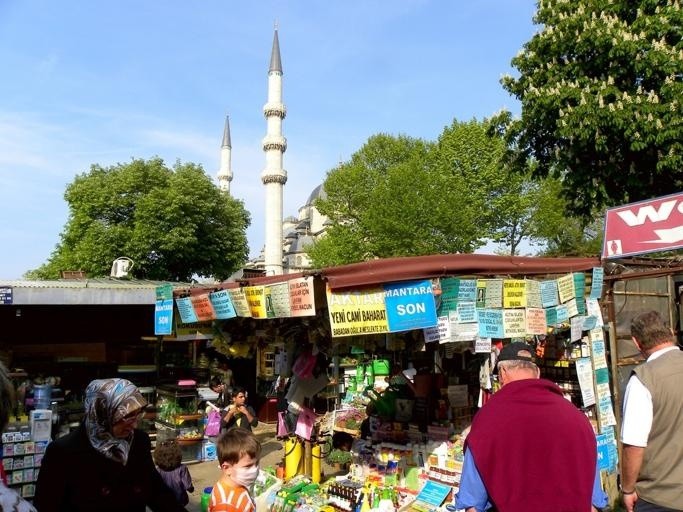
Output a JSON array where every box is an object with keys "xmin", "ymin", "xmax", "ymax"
[{"xmin": 409, "ymin": 480, "xmax": 452, "ymax": 512}]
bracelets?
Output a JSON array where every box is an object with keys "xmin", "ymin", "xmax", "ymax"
[{"xmin": 619, "ymin": 484, "xmax": 636, "ymax": 494}]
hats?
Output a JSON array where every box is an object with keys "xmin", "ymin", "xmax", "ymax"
[{"xmin": 493, "ymin": 342, "xmax": 540, "ymax": 374}]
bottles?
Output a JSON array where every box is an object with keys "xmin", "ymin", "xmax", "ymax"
[
  {"xmin": 375, "ymin": 438, "xmax": 436, "ymax": 467},
  {"xmin": 421, "ymin": 435, "xmax": 465, "ymax": 489},
  {"xmin": 325, "ymin": 467, "xmax": 407, "ymax": 512}
]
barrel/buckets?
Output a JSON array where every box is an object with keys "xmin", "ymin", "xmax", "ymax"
[{"xmin": 33, "ymin": 384, "xmax": 50, "ymax": 410}]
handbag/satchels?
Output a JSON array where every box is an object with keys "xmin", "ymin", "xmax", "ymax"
[
  {"xmin": 308, "ymin": 322, "xmax": 330, "ymax": 344},
  {"xmin": 385, "ymin": 369, "xmax": 416, "ymax": 393},
  {"xmin": 281, "ymin": 413, "xmax": 296, "ymax": 432},
  {"xmin": 294, "ymin": 407, "xmax": 318, "ymax": 441},
  {"xmin": 374, "ymin": 387, "xmax": 398, "ymax": 419},
  {"xmin": 205, "ymin": 412, "xmax": 221, "ymax": 436},
  {"xmin": 313, "ymin": 351, "xmax": 332, "ymax": 373},
  {"xmin": 277, "ymin": 412, "xmax": 291, "ymax": 437}
]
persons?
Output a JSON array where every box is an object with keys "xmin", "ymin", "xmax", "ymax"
[
  {"xmin": 206, "ymin": 426, "xmax": 262, "ymax": 512},
  {"xmin": 431, "ymin": 277, "xmax": 439, "ymax": 290},
  {"xmin": 478, "ymin": 289, "xmax": 483, "ymax": 301},
  {"xmin": 33, "ymin": 377, "xmax": 189, "ymax": 511},
  {"xmin": 209, "ymin": 376, "xmax": 235, "ymax": 410},
  {"xmin": 1, "ymin": 362, "xmax": 38, "ymax": 512},
  {"xmin": 275, "ymin": 377, "xmax": 286, "ymax": 392},
  {"xmin": 215, "ymin": 361, "xmax": 235, "ymax": 387},
  {"xmin": 220, "ymin": 386, "xmax": 259, "ymax": 433},
  {"xmin": 152, "ymin": 438, "xmax": 194, "ymax": 507},
  {"xmin": 332, "ymin": 430, "xmax": 376, "ymax": 471},
  {"xmin": 618, "ymin": 309, "xmax": 683, "ymax": 512},
  {"xmin": 454, "ymin": 341, "xmax": 609, "ymax": 512}
]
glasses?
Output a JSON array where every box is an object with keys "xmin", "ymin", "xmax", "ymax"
[{"xmin": 122, "ymin": 413, "xmax": 142, "ymax": 425}]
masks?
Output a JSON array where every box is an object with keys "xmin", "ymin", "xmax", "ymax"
[{"xmin": 228, "ymin": 464, "xmax": 260, "ymax": 487}]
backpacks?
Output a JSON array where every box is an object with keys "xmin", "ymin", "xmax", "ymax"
[{"xmin": 292, "ymin": 348, "xmax": 317, "ymax": 380}]
